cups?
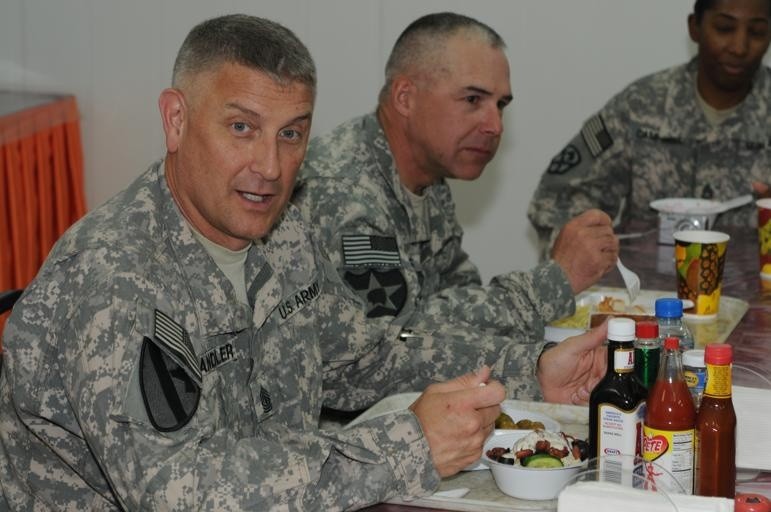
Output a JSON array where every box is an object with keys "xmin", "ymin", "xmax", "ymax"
[
  {"xmin": 673, "ymin": 229, "xmax": 730, "ymax": 316},
  {"xmin": 756, "ymin": 197, "xmax": 771, "ymax": 280}
]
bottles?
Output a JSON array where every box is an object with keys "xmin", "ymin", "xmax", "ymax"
[{"xmin": 587, "ymin": 298, "xmax": 737, "ymax": 498}]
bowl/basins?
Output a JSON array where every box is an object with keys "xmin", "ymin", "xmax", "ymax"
[
  {"xmin": 650, "ymin": 196, "xmax": 722, "ymax": 245},
  {"xmin": 480, "ymin": 430, "xmax": 588, "ymax": 501}
]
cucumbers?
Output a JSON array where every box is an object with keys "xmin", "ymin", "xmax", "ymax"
[{"xmin": 522, "ymin": 454, "xmax": 563, "ymax": 469}]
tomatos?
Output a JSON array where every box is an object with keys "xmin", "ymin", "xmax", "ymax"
[{"xmin": 735, "ymin": 493, "xmax": 771, "ymax": 512}]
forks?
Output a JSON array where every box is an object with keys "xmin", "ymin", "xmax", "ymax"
[{"xmin": 616, "ymin": 256, "xmax": 641, "ymax": 302}]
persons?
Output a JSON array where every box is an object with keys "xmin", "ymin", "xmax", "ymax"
[
  {"xmin": 0, "ymin": 14, "xmax": 616, "ymax": 512},
  {"xmin": 288, "ymin": 12, "xmax": 621, "ymax": 346},
  {"xmin": 525, "ymin": 0, "xmax": 771, "ymax": 245}
]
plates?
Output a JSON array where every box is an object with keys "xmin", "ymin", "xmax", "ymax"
[
  {"xmin": 337, "ymin": 402, "xmax": 564, "ymax": 474},
  {"xmin": 544, "ymin": 292, "xmax": 696, "ymax": 342}
]
